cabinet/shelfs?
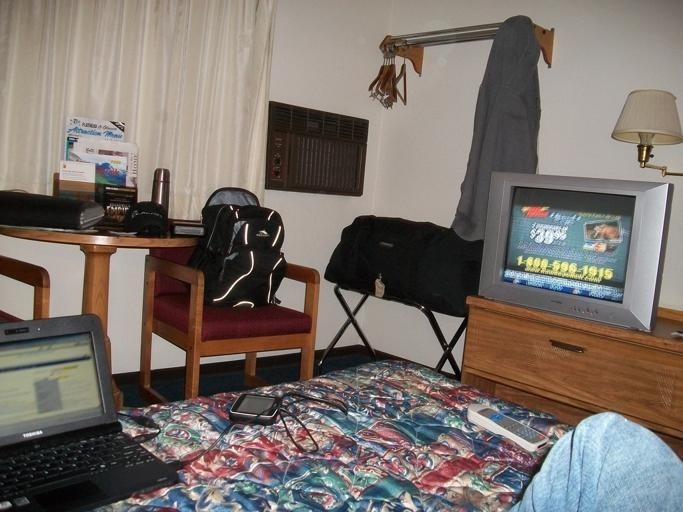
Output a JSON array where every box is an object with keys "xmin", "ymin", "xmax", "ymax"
[{"xmin": 459, "ymin": 293, "xmax": 681, "ymax": 460}]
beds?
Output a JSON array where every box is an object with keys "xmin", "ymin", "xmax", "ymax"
[{"xmin": 85, "ymin": 359, "xmax": 575, "ymax": 512}]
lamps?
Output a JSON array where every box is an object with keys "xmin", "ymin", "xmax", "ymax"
[{"xmin": 609, "ymin": 89, "xmax": 683, "ymax": 175}]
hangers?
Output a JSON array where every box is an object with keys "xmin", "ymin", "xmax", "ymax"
[{"xmin": 366, "ymin": 40, "xmax": 408, "ymax": 109}]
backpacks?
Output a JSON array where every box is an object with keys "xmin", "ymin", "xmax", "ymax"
[{"xmin": 187, "ymin": 186, "xmax": 287, "ymax": 309}]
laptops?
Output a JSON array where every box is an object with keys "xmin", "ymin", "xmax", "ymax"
[{"xmin": 0, "ymin": 314, "xmax": 179, "ymax": 512}]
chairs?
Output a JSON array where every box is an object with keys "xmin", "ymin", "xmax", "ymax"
[
  {"xmin": 139, "ymin": 219, "xmax": 320, "ymax": 405},
  {"xmin": 316, "ymin": 215, "xmax": 485, "ymax": 374}
]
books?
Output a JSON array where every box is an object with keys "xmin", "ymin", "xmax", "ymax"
[
  {"xmin": 169, "ymin": 219, "xmax": 205, "ymax": 238},
  {"xmin": 58, "ymin": 115, "xmax": 138, "ymax": 203}
]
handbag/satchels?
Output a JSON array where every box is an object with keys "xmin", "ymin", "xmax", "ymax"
[{"xmin": 325, "ymin": 214, "xmax": 484, "ymax": 318}]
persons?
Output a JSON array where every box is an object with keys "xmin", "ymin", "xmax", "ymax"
[{"xmin": 507, "ymin": 410, "xmax": 683, "ymax": 512}]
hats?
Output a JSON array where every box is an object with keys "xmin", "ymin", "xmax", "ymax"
[{"xmin": 124, "ymin": 202, "xmax": 167, "ymax": 234}]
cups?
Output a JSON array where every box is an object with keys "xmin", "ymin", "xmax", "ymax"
[{"xmin": 152, "ymin": 168, "xmax": 170, "ymax": 210}]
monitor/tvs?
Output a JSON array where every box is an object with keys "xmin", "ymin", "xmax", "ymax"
[{"xmin": 478, "ymin": 171, "xmax": 675, "ymax": 333}]
